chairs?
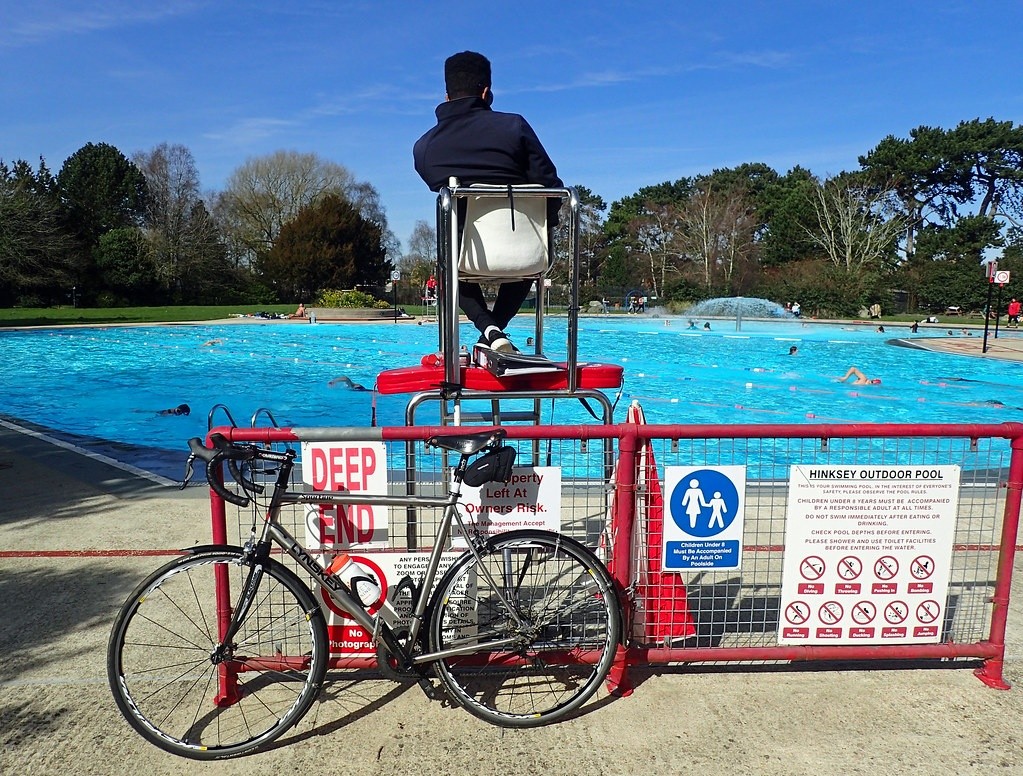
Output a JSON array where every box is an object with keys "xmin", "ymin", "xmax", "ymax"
[{"xmin": 435, "ymin": 177, "xmax": 579, "ymax": 393}]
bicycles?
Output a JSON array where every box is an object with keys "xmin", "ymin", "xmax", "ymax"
[{"xmin": 105, "ymin": 436, "xmax": 628, "ymax": 761}]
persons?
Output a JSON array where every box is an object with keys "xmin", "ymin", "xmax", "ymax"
[
  {"xmin": 427, "ymin": 275, "xmax": 438, "ymax": 298},
  {"xmin": 910, "ymin": 320, "xmax": 918, "ymax": 333},
  {"xmin": 786, "ymin": 301, "xmax": 800, "ymax": 317},
  {"xmin": 790, "ymin": 346, "xmax": 798, "ymax": 356},
  {"xmin": 948, "ymin": 330, "xmax": 952, "ymax": 336},
  {"xmin": 628, "ymin": 299, "xmax": 637, "ymax": 314},
  {"xmin": 412, "ymin": 50, "xmax": 565, "ymax": 352},
  {"xmin": 686, "ymin": 322, "xmax": 711, "ymax": 330},
  {"xmin": 636, "ymin": 296, "xmax": 644, "ymax": 314},
  {"xmin": 839, "ymin": 367, "xmax": 881, "ymax": 385},
  {"xmin": 1006, "ymin": 298, "xmax": 1022, "ymax": 328},
  {"xmin": 601, "ymin": 298, "xmax": 610, "ymax": 313},
  {"xmin": 962, "ymin": 328, "xmax": 973, "ymax": 336},
  {"xmin": 876, "ymin": 326, "xmax": 884, "ymax": 332},
  {"xmin": 398, "ymin": 307, "xmax": 411, "ymax": 318},
  {"xmin": 983, "ymin": 305, "xmax": 996, "ymax": 319},
  {"xmin": 288, "ymin": 304, "xmax": 306, "ymax": 318}
]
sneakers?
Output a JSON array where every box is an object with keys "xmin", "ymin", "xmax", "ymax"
[
  {"xmin": 474, "ymin": 334, "xmax": 491, "ymax": 350},
  {"xmin": 488, "ymin": 329, "xmax": 523, "ymax": 355}
]
